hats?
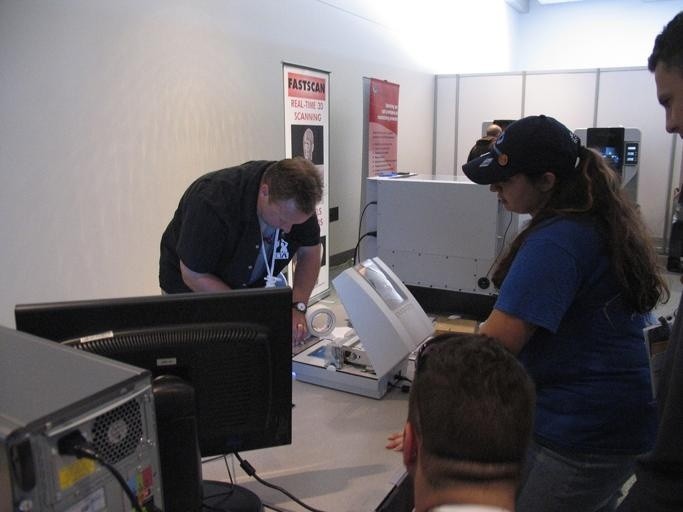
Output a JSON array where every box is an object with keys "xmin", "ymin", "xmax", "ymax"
[{"xmin": 460, "ymin": 114, "xmax": 582, "ymax": 185}]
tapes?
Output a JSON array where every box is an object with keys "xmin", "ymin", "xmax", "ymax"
[{"xmin": 305, "ymin": 304, "xmax": 336, "ymax": 338}]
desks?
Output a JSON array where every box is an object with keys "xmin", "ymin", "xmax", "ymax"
[{"xmin": 200, "ymin": 326, "xmax": 415, "ymax": 511}]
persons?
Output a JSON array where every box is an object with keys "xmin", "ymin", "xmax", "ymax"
[
  {"xmin": 384, "ymin": 115, "xmax": 669, "ymax": 512},
  {"xmin": 159, "ymin": 155, "xmax": 324, "ymax": 346},
  {"xmin": 614, "ymin": 11, "xmax": 683, "ymax": 512},
  {"xmin": 403, "ymin": 333, "xmax": 536, "ymax": 512},
  {"xmin": 467, "ymin": 124, "xmax": 502, "ymax": 162}
]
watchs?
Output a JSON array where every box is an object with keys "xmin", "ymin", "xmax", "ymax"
[{"xmin": 292, "ymin": 301, "xmax": 307, "ymax": 313}]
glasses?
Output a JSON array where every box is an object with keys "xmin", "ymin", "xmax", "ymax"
[{"xmin": 413, "ymin": 331, "xmax": 477, "ymax": 435}]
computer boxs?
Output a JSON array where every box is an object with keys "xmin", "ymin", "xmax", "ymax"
[
  {"xmin": 374, "ymin": 175, "xmax": 519, "ymax": 296},
  {"xmin": 1, "ymin": 324, "xmax": 166, "ymax": 512}
]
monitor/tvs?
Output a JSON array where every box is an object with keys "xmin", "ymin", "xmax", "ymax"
[{"xmin": 14, "ymin": 285, "xmax": 293, "ymax": 512}]
